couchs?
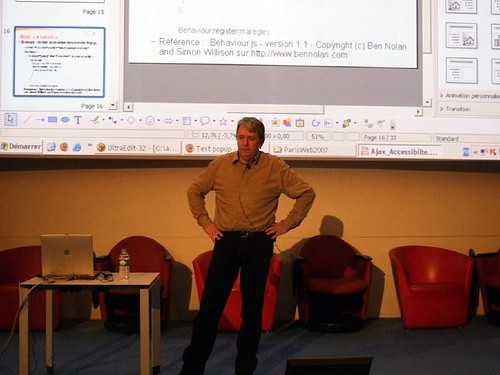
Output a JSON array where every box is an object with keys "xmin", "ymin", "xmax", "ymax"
[
  {"xmin": 192, "ymin": 250, "xmax": 283, "ymax": 335},
  {"xmin": 292, "ymin": 235, "xmax": 372, "ymax": 333},
  {"xmin": 0, "ymin": 246, "xmax": 64, "ymax": 330},
  {"xmin": 389, "ymin": 246, "xmax": 475, "ymax": 332},
  {"xmin": 477, "ymin": 248, "xmax": 500, "ymax": 326},
  {"xmin": 96, "ymin": 236, "xmax": 175, "ymax": 332}
]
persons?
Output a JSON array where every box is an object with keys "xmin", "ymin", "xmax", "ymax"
[{"xmin": 179, "ymin": 117, "xmax": 317, "ymax": 375}]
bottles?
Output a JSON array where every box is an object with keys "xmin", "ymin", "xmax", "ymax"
[{"xmin": 119, "ymin": 249, "xmax": 130, "ymax": 281}]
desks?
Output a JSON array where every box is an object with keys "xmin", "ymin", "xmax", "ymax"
[{"xmin": 19, "ymin": 272, "xmax": 161, "ymax": 375}]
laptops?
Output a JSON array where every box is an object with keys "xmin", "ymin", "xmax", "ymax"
[{"xmin": 40, "ymin": 234, "xmax": 102, "ymax": 279}]
